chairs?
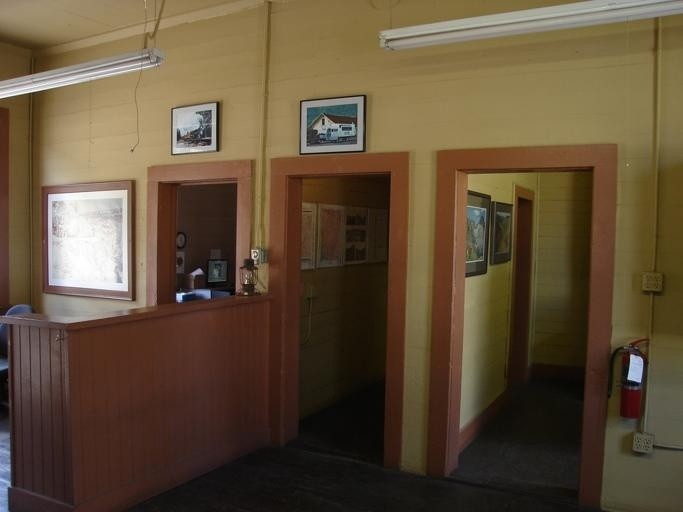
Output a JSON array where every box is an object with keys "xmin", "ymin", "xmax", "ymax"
[{"xmin": 0, "ymin": 301, "xmax": 37, "ymax": 414}]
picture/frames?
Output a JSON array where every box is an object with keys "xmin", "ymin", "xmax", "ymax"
[
  {"xmin": 461, "ymin": 188, "xmax": 515, "ymax": 278},
  {"xmin": 300, "ymin": 201, "xmax": 386, "ymax": 269},
  {"xmin": 38, "ymin": 178, "xmax": 135, "ymax": 302},
  {"xmin": 205, "ymin": 257, "xmax": 230, "ymax": 286},
  {"xmin": 297, "ymin": 92, "xmax": 368, "ymax": 154},
  {"xmin": 168, "ymin": 99, "xmax": 219, "ymax": 156}
]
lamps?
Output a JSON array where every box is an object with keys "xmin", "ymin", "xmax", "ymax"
[
  {"xmin": 238, "ymin": 256, "xmax": 260, "ymax": 297},
  {"xmin": 0, "ymin": 0, "xmax": 167, "ymax": 101},
  {"xmin": 378, "ymin": 0, "xmax": 682, "ymax": 53}
]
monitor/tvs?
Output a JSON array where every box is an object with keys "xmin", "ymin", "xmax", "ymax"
[{"xmin": 207, "ymin": 259, "xmax": 228, "ymax": 285}]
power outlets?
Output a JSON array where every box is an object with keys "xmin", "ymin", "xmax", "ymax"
[
  {"xmin": 631, "ymin": 431, "xmax": 654, "ymax": 454},
  {"xmin": 640, "ymin": 271, "xmax": 663, "ymax": 293}
]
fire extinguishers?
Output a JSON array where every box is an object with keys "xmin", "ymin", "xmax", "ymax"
[{"xmin": 606, "ymin": 338, "xmax": 648, "ymax": 419}]
